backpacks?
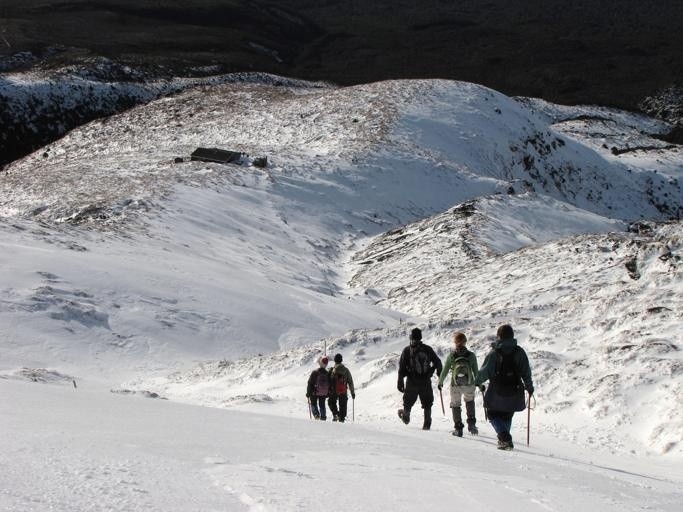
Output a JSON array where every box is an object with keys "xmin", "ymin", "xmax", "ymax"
[
  {"xmin": 452, "ymin": 351, "xmax": 472, "ymax": 387},
  {"xmin": 495, "ymin": 348, "xmax": 521, "ymax": 396},
  {"xmin": 331, "ymin": 368, "xmax": 347, "ymax": 395},
  {"xmin": 314, "ymin": 371, "xmax": 329, "ymax": 397},
  {"xmin": 408, "ymin": 345, "xmax": 433, "ymax": 383}
]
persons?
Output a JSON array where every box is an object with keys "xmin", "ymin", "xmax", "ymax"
[
  {"xmin": 438, "ymin": 333, "xmax": 485, "ymax": 437},
  {"xmin": 306, "ymin": 358, "xmax": 330, "ymax": 420},
  {"xmin": 475, "ymin": 325, "xmax": 534, "ymax": 450},
  {"xmin": 328, "ymin": 354, "xmax": 355, "ymax": 421},
  {"xmin": 397, "ymin": 329, "xmax": 442, "ymax": 430}
]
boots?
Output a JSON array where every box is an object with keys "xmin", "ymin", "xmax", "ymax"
[
  {"xmin": 453, "ymin": 425, "xmax": 462, "ymax": 436},
  {"xmin": 467, "ymin": 419, "xmax": 477, "ymax": 434}
]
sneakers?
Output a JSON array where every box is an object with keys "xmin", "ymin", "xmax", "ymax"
[
  {"xmin": 332, "ymin": 411, "xmax": 345, "ymax": 422},
  {"xmin": 398, "ymin": 409, "xmax": 409, "ymax": 424},
  {"xmin": 422, "ymin": 419, "xmax": 430, "ymax": 430},
  {"xmin": 314, "ymin": 413, "xmax": 326, "ymax": 420},
  {"xmin": 496, "ymin": 434, "xmax": 514, "ymax": 450}
]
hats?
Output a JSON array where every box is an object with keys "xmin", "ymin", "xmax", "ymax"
[
  {"xmin": 334, "ymin": 354, "xmax": 342, "ymax": 363},
  {"xmin": 410, "ymin": 328, "xmax": 422, "ymax": 340},
  {"xmin": 497, "ymin": 325, "xmax": 514, "ymax": 338},
  {"xmin": 454, "ymin": 334, "xmax": 466, "ymax": 344},
  {"xmin": 320, "ymin": 357, "xmax": 328, "ymax": 368}
]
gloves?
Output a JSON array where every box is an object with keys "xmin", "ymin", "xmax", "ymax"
[
  {"xmin": 438, "ymin": 385, "xmax": 443, "ymax": 389},
  {"xmin": 398, "ymin": 380, "xmax": 405, "ymax": 392},
  {"xmin": 480, "ymin": 385, "xmax": 486, "ymax": 391}
]
